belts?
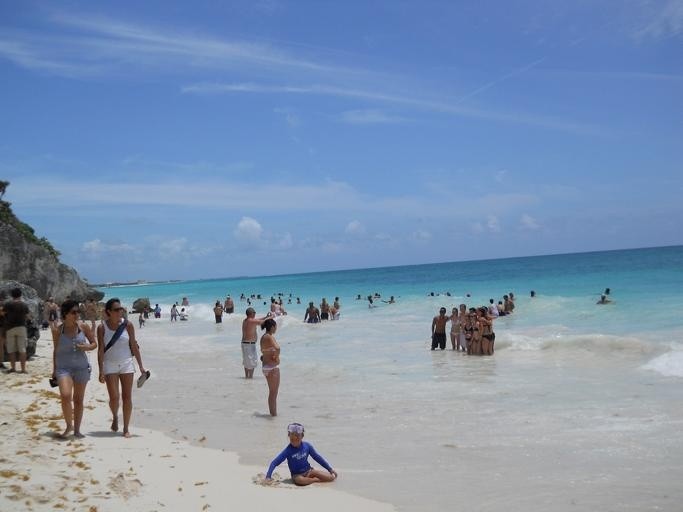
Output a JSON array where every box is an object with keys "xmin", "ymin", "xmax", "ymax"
[{"xmin": 242, "ymin": 341, "xmax": 256, "ymax": 344}]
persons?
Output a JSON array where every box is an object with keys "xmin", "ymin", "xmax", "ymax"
[
  {"xmin": 213, "ymin": 294, "xmax": 234, "ymax": 323},
  {"xmin": 258, "ymin": 318, "xmax": 280, "ymax": 417},
  {"xmin": 430, "ymin": 303, "xmax": 498, "ymax": 356},
  {"xmin": 240, "ymin": 292, "xmax": 301, "ymax": 320},
  {"xmin": 531, "ymin": 291, "xmax": 536, "ymax": 297},
  {"xmin": 356, "ymin": 292, "xmax": 395, "ymax": 309},
  {"xmin": 96, "ymin": 297, "xmax": 146, "ymax": 439},
  {"xmin": 1, "ymin": 287, "xmax": 39, "ymax": 375},
  {"xmin": 597, "ymin": 287, "xmax": 613, "ymax": 304},
  {"xmin": 304, "ymin": 296, "xmax": 340, "ymax": 323},
  {"xmin": 171, "ymin": 297, "xmax": 190, "ymax": 322},
  {"xmin": 428, "ymin": 292, "xmax": 471, "ymax": 297},
  {"xmin": 50, "ymin": 299, "xmax": 97, "ymax": 439},
  {"xmin": 488, "ymin": 293, "xmax": 517, "ymax": 316},
  {"xmin": 139, "ymin": 303, "xmax": 161, "ymax": 327},
  {"xmin": 38, "ymin": 297, "xmax": 58, "ymax": 330},
  {"xmin": 66, "ymin": 295, "xmax": 94, "ymax": 323},
  {"xmin": 265, "ymin": 422, "xmax": 338, "ymax": 486}
]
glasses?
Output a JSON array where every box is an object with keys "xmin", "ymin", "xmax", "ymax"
[
  {"xmin": 68, "ymin": 310, "xmax": 81, "ymax": 315},
  {"xmin": 111, "ymin": 308, "xmax": 123, "ymax": 313},
  {"xmin": 288, "ymin": 424, "xmax": 304, "ymax": 434}
]
